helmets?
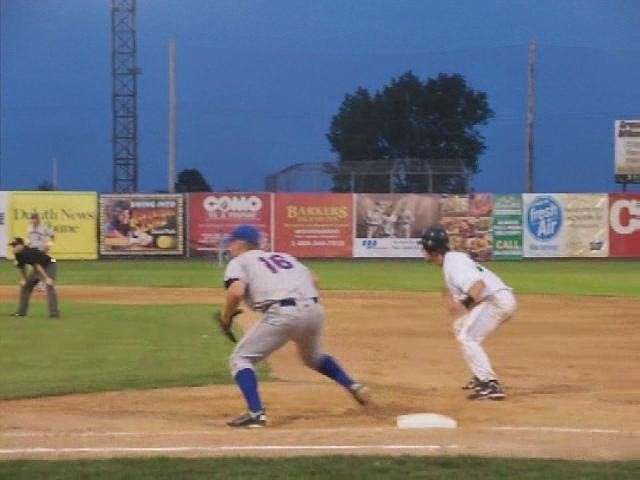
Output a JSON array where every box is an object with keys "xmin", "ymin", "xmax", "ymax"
[{"xmin": 417, "ymin": 225, "xmax": 450, "ymax": 251}]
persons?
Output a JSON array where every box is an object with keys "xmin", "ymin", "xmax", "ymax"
[
  {"xmin": 103, "ymin": 201, "xmax": 136, "ymax": 248},
  {"xmin": 29, "ymin": 212, "xmax": 55, "ymax": 291},
  {"xmin": 423, "ymin": 224, "xmax": 516, "ymax": 399},
  {"xmin": 9, "ymin": 237, "xmax": 57, "ymax": 320},
  {"xmin": 223, "ymin": 226, "xmax": 370, "ymax": 428}
]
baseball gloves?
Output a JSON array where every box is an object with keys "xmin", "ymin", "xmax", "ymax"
[{"xmin": 212, "ymin": 309, "xmax": 243, "ymax": 343}]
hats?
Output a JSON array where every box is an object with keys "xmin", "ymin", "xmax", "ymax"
[
  {"xmin": 223, "ymin": 226, "xmax": 259, "ymax": 245},
  {"xmin": 8, "ymin": 236, "xmax": 24, "ymax": 246}
]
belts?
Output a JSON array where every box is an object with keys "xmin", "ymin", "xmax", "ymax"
[{"xmin": 280, "ymin": 297, "xmax": 318, "ymax": 305}]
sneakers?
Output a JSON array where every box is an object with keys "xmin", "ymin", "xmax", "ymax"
[
  {"xmin": 348, "ymin": 382, "xmax": 371, "ymax": 404},
  {"xmin": 462, "ymin": 375, "xmax": 506, "ymax": 401},
  {"xmin": 225, "ymin": 410, "xmax": 267, "ymax": 428}
]
